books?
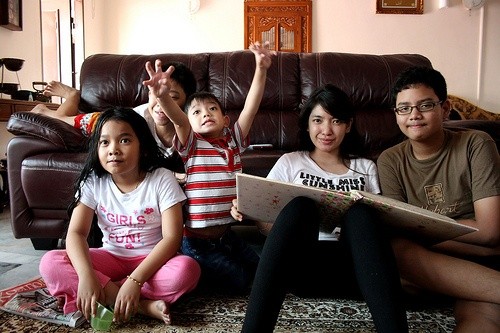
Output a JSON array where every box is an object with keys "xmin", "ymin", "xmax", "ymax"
[
  {"xmin": 235, "ymin": 172, "xmax": 479, "ymax": 248},
  {"xmin": 261, "ymin": 26, "xmax": 295, "ymax": 49}
]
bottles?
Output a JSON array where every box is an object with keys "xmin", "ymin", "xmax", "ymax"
[{"xmin": 28, "ymin": 91, "xmax": 33, "ymax": 101}]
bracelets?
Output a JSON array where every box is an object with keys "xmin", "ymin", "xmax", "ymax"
[{"xmin": 126, "ymin": 276, "xmax": 144, "ymax": 288}]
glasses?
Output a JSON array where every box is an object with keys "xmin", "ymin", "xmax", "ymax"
[{"xmin": 394, "ymin": 99, "xmax": 442, "ymax": 116}]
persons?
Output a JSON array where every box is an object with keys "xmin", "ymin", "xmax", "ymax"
[
  {"xmin": 231, "ymin": 84, "xmax": 409, "ymax": 332},
  {"xmin": 377, "ymin": 65, "xmax": 500, "ymax": 333},
  {"xmin": 143, "ymin": 41, "xmax": 277, "ymax": 292},
  {"xmin": 40, "ymin": 105, "xmax": 201, "ymax": 325},
  {"xmin": 31, "ymin": 61, "xmax": 197, "ymax": 160}
]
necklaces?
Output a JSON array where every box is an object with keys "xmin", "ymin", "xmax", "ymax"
[{"xmin": 134, "ymin": 173, "xmax": 141, "ymax": 188}]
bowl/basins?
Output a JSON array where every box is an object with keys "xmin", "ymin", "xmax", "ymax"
[{"xmin": 90, "ymin": 301, "xmax": 114, "ymax": 331}]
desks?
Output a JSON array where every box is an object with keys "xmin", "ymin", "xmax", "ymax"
[{"xmin": 0, "ymin": 98, "xmax": 60, "ymax": 122}]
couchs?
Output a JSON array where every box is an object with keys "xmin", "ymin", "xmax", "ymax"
[{"xmin": 5, "ymin": 52, "xmax": 500, "ymax": 250}]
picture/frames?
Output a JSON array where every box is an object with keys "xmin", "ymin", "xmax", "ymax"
[
  {"xmin": 376, "ymin": 0, "xmax": 424, "ymax": 15},
  {"xmin": 0, "ymin": 0, "xmax": 23, "ymax": 31}
]
